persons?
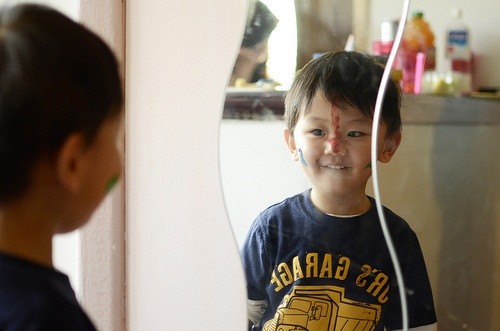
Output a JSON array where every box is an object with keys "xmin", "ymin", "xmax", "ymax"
[
  {"xmin": 0, "ymin": 2, "xmax": 125, "ymax": 331},
  {"xmin": 242, "ymin": 50, "xmax": 438, "ymax": 331}
]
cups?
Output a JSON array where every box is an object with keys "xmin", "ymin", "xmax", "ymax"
[
  {"xmin": 373, "ymin": 41, "xmax": 399, "ymax": 69},
  {"xmin": 399, "ymin": 51, "xmax": 425, "ymax": 96}
]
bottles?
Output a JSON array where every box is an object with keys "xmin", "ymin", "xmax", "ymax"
[
  {"xmin": 401, "ymin": 12, "xmax": 436, "ymax": 95},
  {"xmin": 442, "ymin": 32, "xmax": 472, "ymax": 93}
]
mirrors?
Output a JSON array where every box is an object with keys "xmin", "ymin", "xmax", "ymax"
[{"xmin": 217, "ymin": 0, "xmax": 500, "ymax": 331}]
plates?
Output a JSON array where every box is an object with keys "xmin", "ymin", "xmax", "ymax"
[{"xmin": 226, "ymin": 85, "xmax": 285, "ymax": 99}]
